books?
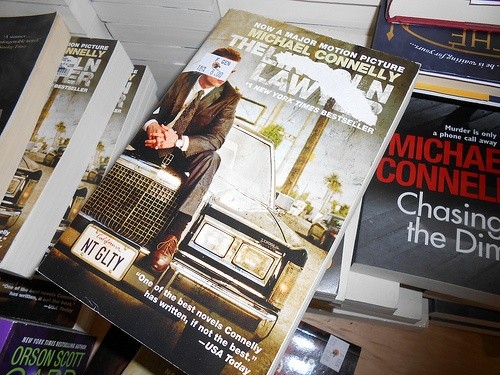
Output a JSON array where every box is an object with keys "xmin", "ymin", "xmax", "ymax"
[{"xmin": 0, "ymin": 0, "xmax": 500, "ymax": 375}]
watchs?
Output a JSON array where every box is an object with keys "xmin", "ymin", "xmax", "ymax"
[{"xmin": 175, "ymin": 133, "xmax": 184, "ymax": 148}]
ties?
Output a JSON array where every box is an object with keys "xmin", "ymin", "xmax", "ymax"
[{"xmin": 158, "ymin": 90, "xmax": 205, "ymax": 159}]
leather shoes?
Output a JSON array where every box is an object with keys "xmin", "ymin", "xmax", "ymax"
[{"xmin": 149, "ymin": 235, "xmax": 179, "ymax": 274}]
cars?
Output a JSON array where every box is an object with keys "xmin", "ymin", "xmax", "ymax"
[
  {"xmin": 55, "ymin": 119, "xmax": 308, "ymax": 374},
  {"xmin": 86, "ymin": 163, "xmax": 107, "ymax": 184},
  {"xmin": 43, "ymin": 145, "xmax": 66, "ymax": 167},
  {"xmin": 307, "ymin": 213, "xmax": 345, "ymax": 253}
]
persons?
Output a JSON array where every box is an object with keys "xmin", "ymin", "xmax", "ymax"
[{"xmin": 129, "ymin": 47, "xmax": 242, "ymax": 273}]
